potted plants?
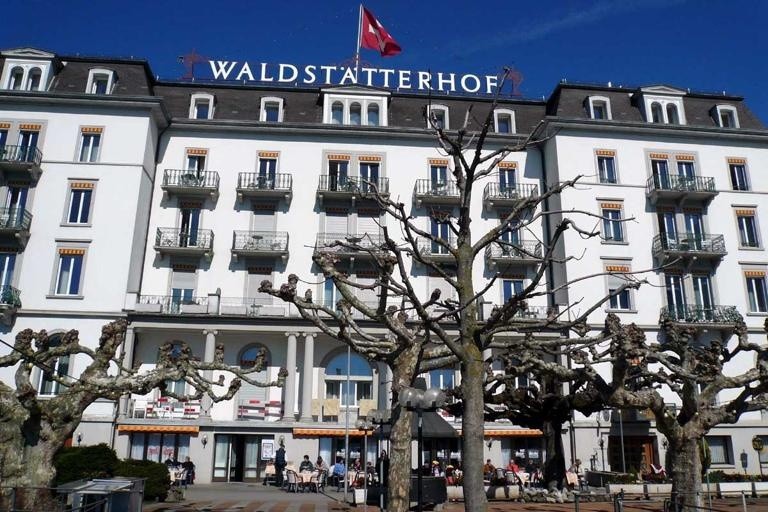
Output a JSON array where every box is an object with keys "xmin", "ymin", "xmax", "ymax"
[{"xmin": 354, "ymin": 477, "xmax": 368, "ymax": 505}]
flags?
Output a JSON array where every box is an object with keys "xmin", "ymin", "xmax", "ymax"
[{"xmin": 359, "ymin": 5, "xmax": 401, "ymax": 58}]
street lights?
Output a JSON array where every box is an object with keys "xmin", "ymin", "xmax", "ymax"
[
  {"xmin": 398, "ymin": 386, "xmax": 446, "ymax": 511},
  {"xmin": 367, "ymin": 408, "xmax": 391, "ymax": 511},
  {"xmin": 355, "ymin": 416, "xmax": 377, "ymax": 511}
]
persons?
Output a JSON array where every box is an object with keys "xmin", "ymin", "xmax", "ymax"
[
  {"xmin": 330, "ymin": 449, "xmax": 389, "ymax": 487},
  {"xmin": 312, "ymin": 455, "xmax": 329, "ymax": 486},
  {"xmin": 274, "ymin": 445, "xmax": 287, "ymax": 486},
  {"xmin": 300, "ymin": 454, "xmax": 312, "ymax": 471},
  {"xmin": 568, "ymin": 458, "xmax": 583, "ymax": 489},
  {"xmin": 506, "ymin": 459, "xmax": 519, "ymax": 472},
  {"xmin": 484, "ymin": 458, "xmax": 496, "ymax": 474},
  {"xmin": 525, "ymin": 460, "xmax": 539, "ymax": 472},
  {"xmin": 182, "ymin": 455, "xmax": 195, "ymax": 474},
  {"xmin": 165, "ymin": 451, "xmax": 182, "ymax": 466},
  {"xmin": 429, "ymin": 456, "xmax": 464, "ymax": 486}
]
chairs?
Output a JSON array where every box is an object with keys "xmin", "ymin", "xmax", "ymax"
[
  {"xmin": 287, "ymin": 467, "xmax": 379, "ymax": 493},
  {"xmin": 483, "ymin": 466, "xmax": 543, "ymax": 485},
  {"xmin": 166, "ymin": 463, "xmax": 195, "ymax": 490}
]
